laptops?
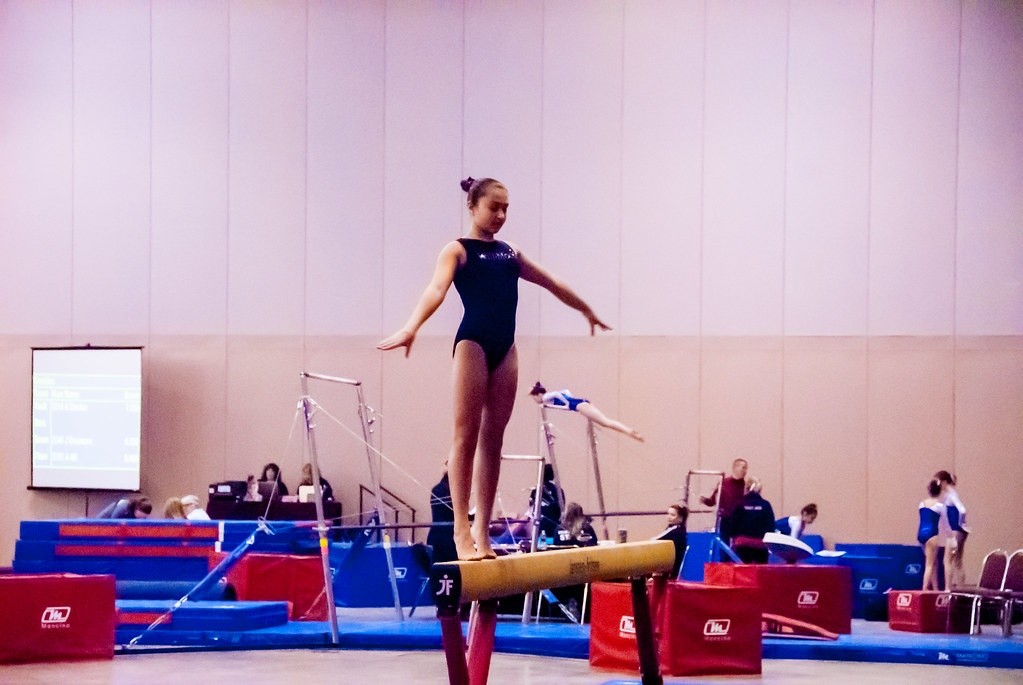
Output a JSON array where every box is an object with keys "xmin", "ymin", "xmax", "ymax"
[
  {"xmin": 299, "ymin": 485, "xmax": 322, "ymax": 502},
  {"xmin": 257, "ymin": 482, "xmax": 278, "ymax": 502}
]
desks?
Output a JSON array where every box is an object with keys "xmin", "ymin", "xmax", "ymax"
[{"xmin": 206, "ymin": 500, "xmax": 341, "ymax": 542}]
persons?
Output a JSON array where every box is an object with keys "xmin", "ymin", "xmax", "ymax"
[
  {"xmin": 96, "ymin": 463, "xmax": 334, "ymax": 519},
  {"xmin": 530, "ymin": 381, "xmax": 643, "ymax": 443},
  {"xmin": 935, "ymin": 471, "xmax": 972, "ymax": 588},
  {"xmin": 917, "ymin": 479, "xmax": 959, "ymax": 591},
  {"xmin": 426, "ymin": 460, "xmax": 818, "ymax": 580},
  {"xmin": 376, "ymin": 177, "xmax": 615, "ymax": 563}
]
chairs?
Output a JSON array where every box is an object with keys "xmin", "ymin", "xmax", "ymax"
[
  {"xmin": 945, "ymin": 548, "xmax": 1023, "ymax": 638},
  {"xmin": 406, "ymin": 539, "xmax": 432, "ymax": 617},
  {"xmin": 535, "ymin": 543, "xmax": 588, "ymax": 628}
]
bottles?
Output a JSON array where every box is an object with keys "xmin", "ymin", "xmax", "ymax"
[{"xmin": 540, "ymin": 530, "xmax": 547, "ymax": 550}]
